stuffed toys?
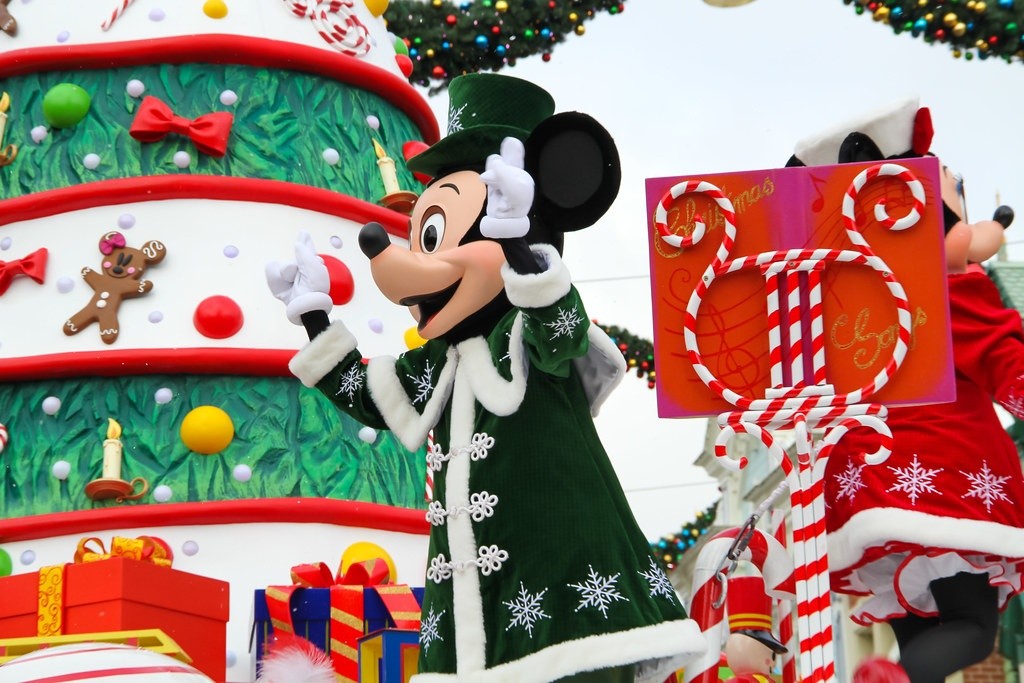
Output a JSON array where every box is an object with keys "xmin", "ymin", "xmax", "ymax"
[
  {"xmin": 688, "ymin": 97, "xmax": 1024, "ymax": 683},
  {"xmin": 266, "ymin": 73, "xmax": 706, "ymax": 683}
]
